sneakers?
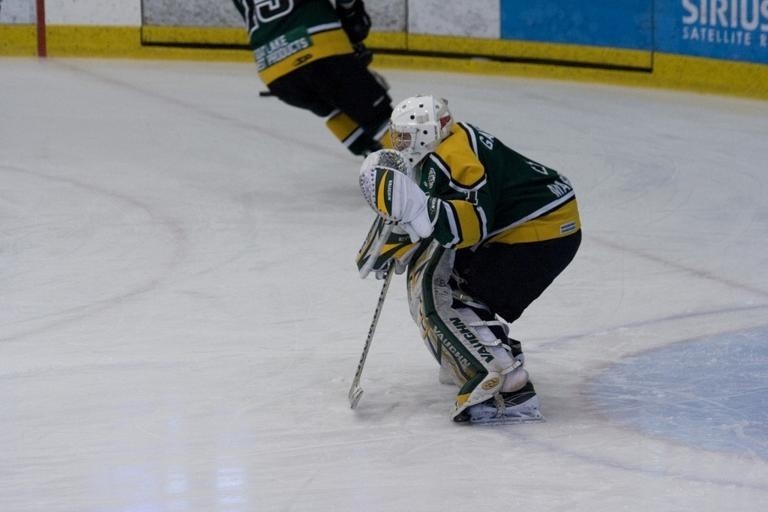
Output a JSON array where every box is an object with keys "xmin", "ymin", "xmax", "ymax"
[{"xmin": 464, "ymin": 381, "xmax": 538, "ymax": 415}]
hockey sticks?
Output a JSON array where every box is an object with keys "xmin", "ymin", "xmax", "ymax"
[{"xmin": 348, "ymin": 258, "xmax": 395, "ymax": 409}]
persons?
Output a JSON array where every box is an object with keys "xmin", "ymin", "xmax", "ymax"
[
  {"xmin": 233, "ymin": 1, "xmax": 398, "ymax": 158},
  {"xmin": 354, "ymin": 91, "xmax": 582, "ymax": 423}
]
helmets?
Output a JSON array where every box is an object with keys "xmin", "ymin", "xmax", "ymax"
[{"xmin": 388, "ymin": 94, "xmax": 453, "ymax": 166}]
